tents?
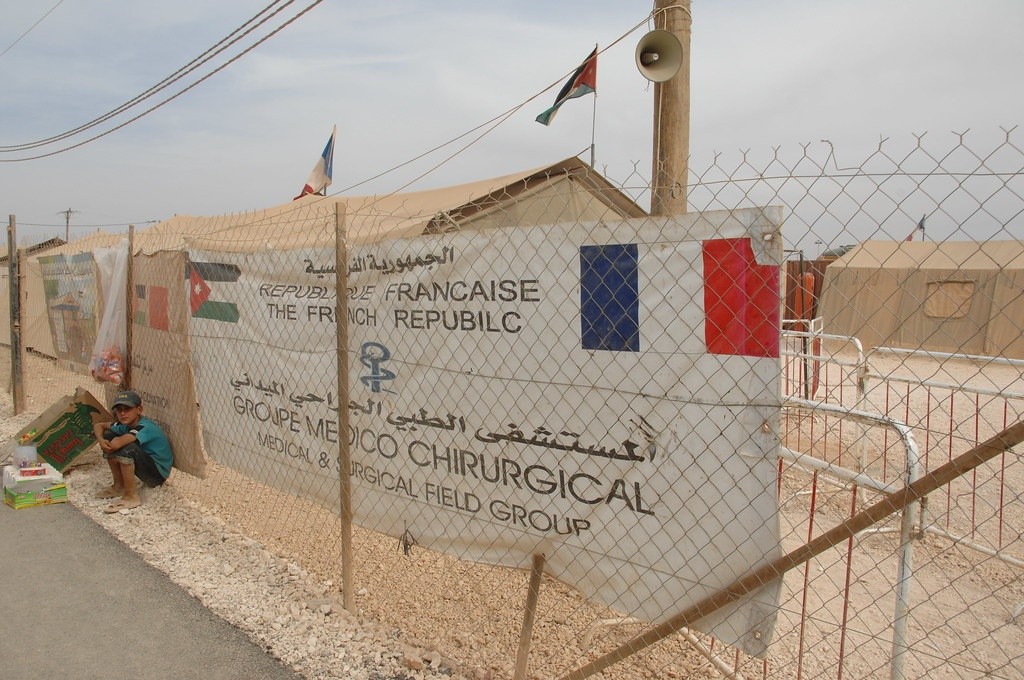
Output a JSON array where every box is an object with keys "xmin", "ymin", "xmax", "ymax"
[
  {"xmin": 0, "ymin": 156, "xmax": 653, "ymax": 381},
  {"xmin": 814, "ymin": 239, "xmax": 1024, "ymax": 362}
]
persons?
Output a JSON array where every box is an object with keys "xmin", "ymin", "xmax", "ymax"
[{"xmin": 93, "ymin": 390, "xmax": 173, "ymax": 512}]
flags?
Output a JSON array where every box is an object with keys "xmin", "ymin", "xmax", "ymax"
[
  {"xmin": 917, "ymin": 218, "xmax": 924, "ymax": 230},
  {"xmin": 293, "ymin": 134, "xmax": 335, "ymax": 200},
  {"xmin": 535, "ymin": 48, "xmax": 596, "ymax": 127}
]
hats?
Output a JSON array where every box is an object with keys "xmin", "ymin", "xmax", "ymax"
[{"xmin": 111, "ymin": 391, "xmax": 141, "ymax": 410}]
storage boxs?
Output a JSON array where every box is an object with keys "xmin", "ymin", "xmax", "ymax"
[
  {"xmin": 13, "ymin": 386, "xmax": 114, "ymax": 475},
  {"xmin": 0, "ymin": 463, "xmax": 68, "ymax": 510}
]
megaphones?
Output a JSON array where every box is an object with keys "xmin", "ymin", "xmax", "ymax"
[{"xmin": 635, "ymin": 29, "xmax": 683, "ymax": 82}]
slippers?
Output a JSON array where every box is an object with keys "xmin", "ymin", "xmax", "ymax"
[
  {"xmin": 96, "ymin": 486, "xmax": 124, "ymax": 498},
  {"xmin": 104, "ymin": 500, "xmax": 141, "ymax": 513}
]
